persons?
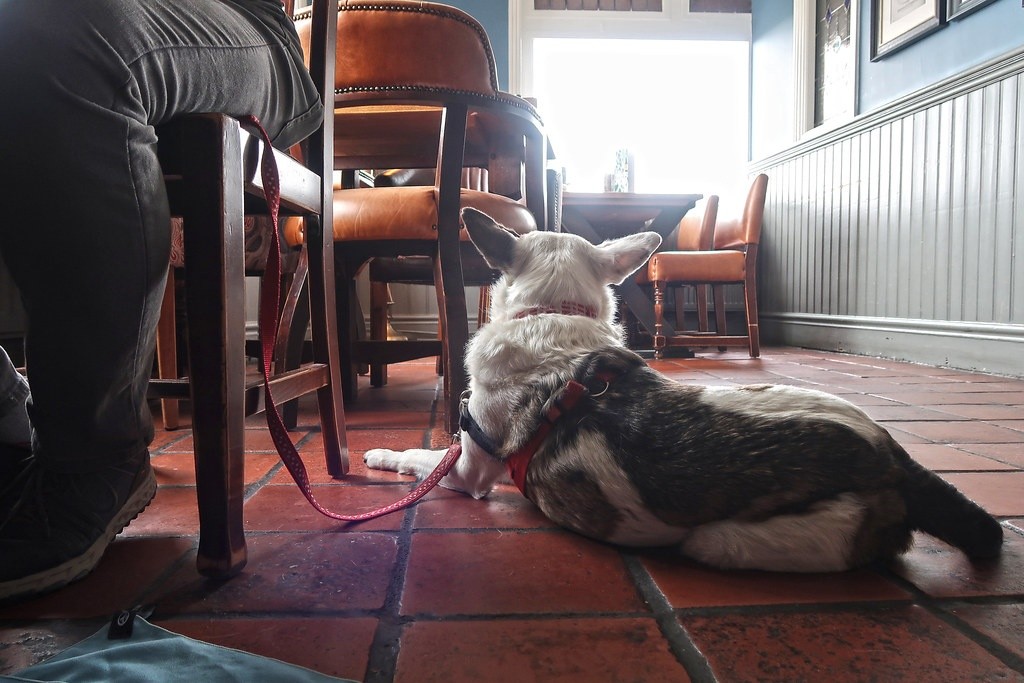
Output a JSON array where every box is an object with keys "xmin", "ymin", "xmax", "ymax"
[{"xmin": 0, "ymin": 1, "xmax": 323, "ymax": 599}]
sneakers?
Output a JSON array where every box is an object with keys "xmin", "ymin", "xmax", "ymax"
[{"xmin": 0, "ymin": 445, "xmax": 157, "ymax": 600}]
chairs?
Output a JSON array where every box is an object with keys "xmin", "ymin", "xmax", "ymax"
[
  {"xmin": 150, "ymin": 0, "xmax": 556, "ymax": 582},
  {"xmin": 640, "ymin": 173, "xmax": 769, "ymax": 361}
]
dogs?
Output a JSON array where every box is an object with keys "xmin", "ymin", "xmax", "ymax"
[{"xmin": 362, "ymin": 207, "xmax": 1005, "ymax": 573}]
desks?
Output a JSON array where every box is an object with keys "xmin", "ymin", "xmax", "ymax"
[{"xmin": 559, "ymin": 192, "xmax": 703, "ymax": 359}]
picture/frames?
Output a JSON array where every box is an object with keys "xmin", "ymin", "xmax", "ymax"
[{"xmin": 870, "ymin": 0, "xmax": 997, "ymax": 65}]
